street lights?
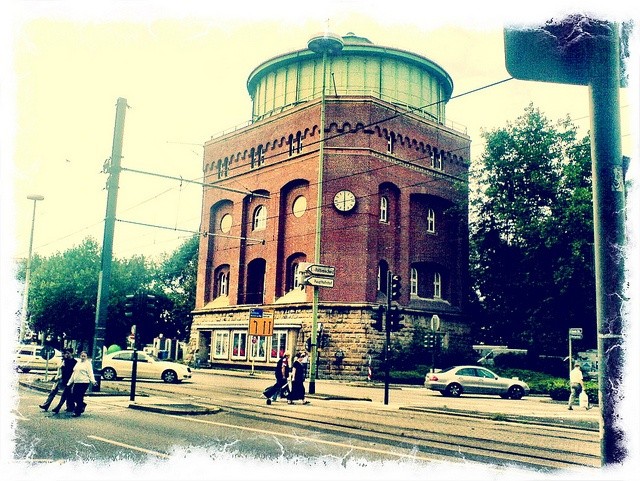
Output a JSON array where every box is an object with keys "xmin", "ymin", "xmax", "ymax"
[{"xmin": 18, "ymin": 193, "xmax": 45, "ymax": 344}]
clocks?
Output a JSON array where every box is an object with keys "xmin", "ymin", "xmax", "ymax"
[{"xmin": 333, "ymin": 189, "xmax": 357, "ymax": 212}]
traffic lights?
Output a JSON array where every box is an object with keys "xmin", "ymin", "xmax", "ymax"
[
  {"xmin": 142, "ymin": 293, "xmax": 155, "ymax": 319},
  {"xmin": 391, "ymin": 306, "xmax": 405, "ymax": 333},
  {"xmin": 370, "ymin": 305, "xmax": 381, "ymax": 332},
  {"xmin": 124, "ymin": 294, "xmax": 134, "ymax": 318},
  {"xmin": 390, "ymin": 273, "xmax": 401, "ymax": 301}
]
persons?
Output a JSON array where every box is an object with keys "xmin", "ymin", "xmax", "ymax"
[
  {"xmin": 272, "ymin": 352, "xmax": 291, "ymax": 402},
  {"xmin": 568, "ymin": 363, "xmax": 591, "ymax": 411},
  {"xmin": 194, "ymin": 349, "xmax": 200, "ymax": 369},
  {"xmin": 67, "ymin": 350, "xmax": 98, "ymax": 416},
  {"xmin": 287, "ymin": 353, "xmax": 310, "ymax": 405},
  {"xmin": 52, "ymin": 347, "xmax": 87, "ymax": 413},
  {"xmin": 38, "ymin": 349, "xmax": 75, "ymax": 412}
]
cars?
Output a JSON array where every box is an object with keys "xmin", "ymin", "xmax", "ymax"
[
  {"xmin": 424, "ymin": 365, "xmax": 529, "ymax": 398},
  {"xmin": 99, "ymin": 350, "xmax": 192, "ymax": 383}
]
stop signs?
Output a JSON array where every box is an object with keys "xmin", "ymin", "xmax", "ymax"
[{"xmin": 130, "ymin": 334, "xmax": 135, "ymax": 339}]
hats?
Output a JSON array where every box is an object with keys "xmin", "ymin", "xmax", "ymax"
[{"xmin": 284, "ymin": 353, "xmax": 290, "ymax": 356}]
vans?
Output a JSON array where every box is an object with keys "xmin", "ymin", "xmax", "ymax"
[
  {"xmin": 17, "ymin": 342, "xmax": 67, "ymax": 371},
  {"xmin": 473, "ymin": 344, "xmax": 599, "ymax": 368}
]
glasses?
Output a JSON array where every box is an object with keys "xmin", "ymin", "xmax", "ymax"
[{"xmin": 287, "ymin": 356, "xmax": 290, "ymax": 359}]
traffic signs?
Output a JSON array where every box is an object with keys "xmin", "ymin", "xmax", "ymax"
[
  {"xmin": 308, "ymin": 277, "xmax": 334, "ymax": 288},
  {"xmin": 571, "ymin": 334, "xmax": 583, "ymax": 339},
  {"xmin": 569, "ymin": 327, "xmax": 582, "ymax": 334},
  {"xmin": 307, "ymin": 264, "xmax": 334, "ymax": 277}
]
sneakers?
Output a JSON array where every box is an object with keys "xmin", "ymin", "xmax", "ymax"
[
  {"xmin": 81, "ymin": 402, "xmax": 87, "ymax": 413},
  {"xmin": 39, "ymin": 404, "xmax": 48, "ymax": 411},
  {"xmin": 288, "ymin": 401, "xmax": 295, "ymax": 405},
  {"xmin": 51, "ymin": 406, "xmax": 60, "ymax": 413},
  {"xmin": 271, "ymin": 399, "xmax": 279, "ymax": 402},
  {"xmin": 72, "ymin": 413, "xmax": 80, "ymax": 417},
  {"xmin": 303, "ymin": 401, "xmax": 310, "ymax": 405},
  {"xmin": 262, "ymin": 393, "xmax": 268, "ymax": 398}
]
